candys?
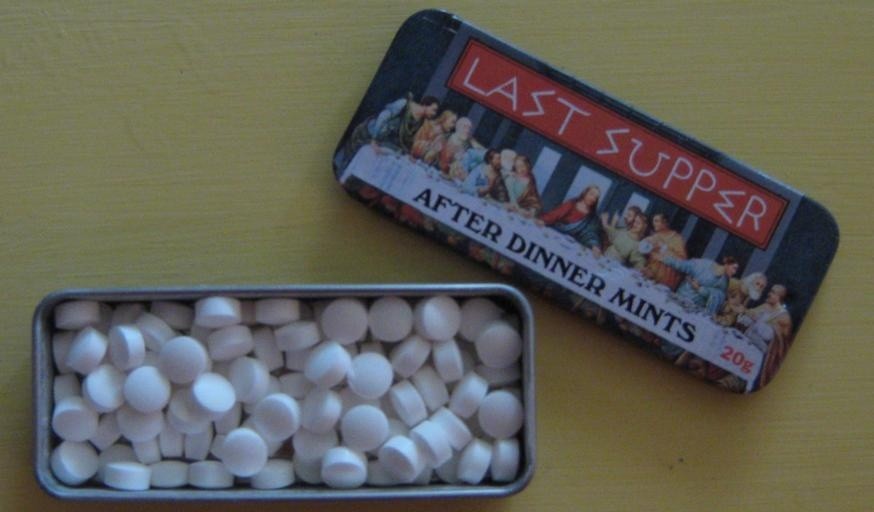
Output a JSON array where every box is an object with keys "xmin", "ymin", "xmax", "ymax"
[{"xmin": 50, "ymin": 294, "xmax": 523, "ymax": 493}]
